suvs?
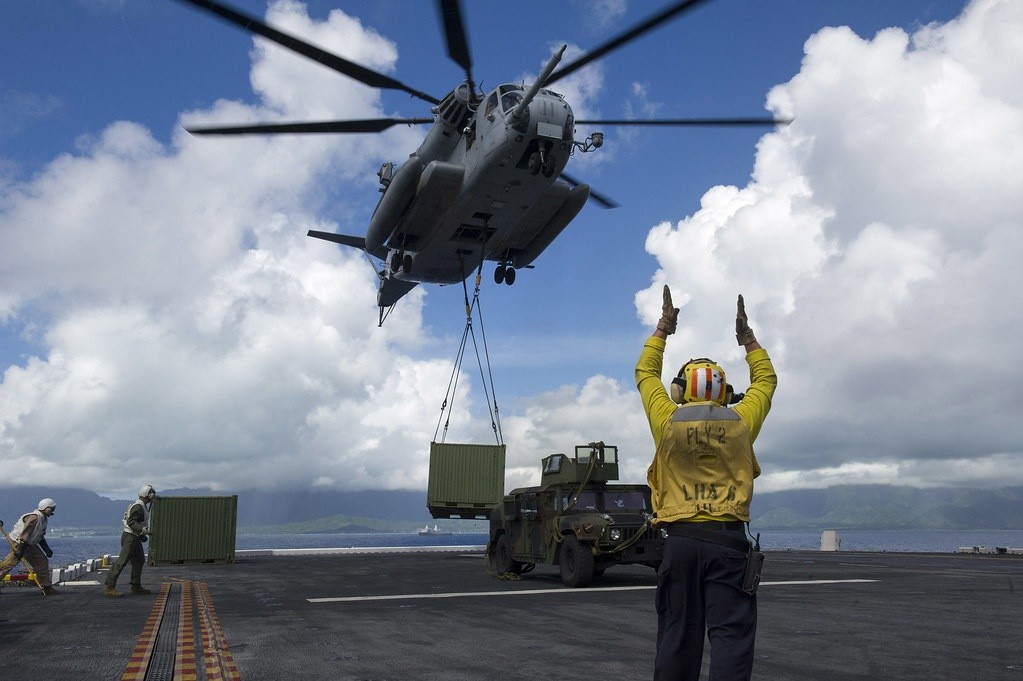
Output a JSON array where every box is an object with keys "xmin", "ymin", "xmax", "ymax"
[{"xmin": 483, "ymin": 441, "xmax": 665, "ymax": 589}]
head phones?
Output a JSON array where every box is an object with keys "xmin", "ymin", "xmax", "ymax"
[
  {"xmin": 45, "ymin": 508, "xmax": 52, "ymax": 516},
  {"xmin": 149, "ymin": 486, "xmax": 155, "ymax": 501},
  {"xmin": 671, "ymin": 357, "xmax": 733, "ymax": 408}
]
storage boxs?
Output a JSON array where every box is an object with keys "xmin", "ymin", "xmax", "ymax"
[
  {"xmin": 426, "ymin": 441, "xmax": 506, "ymax": 520},
  {"xmin": 147, "ymin": 495, "xmax": 237, "ymax": 566}
]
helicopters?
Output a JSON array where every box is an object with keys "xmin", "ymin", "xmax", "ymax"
[{"xmin": 175, "ymin": 1, "xmax": 796, "ymax": 328}]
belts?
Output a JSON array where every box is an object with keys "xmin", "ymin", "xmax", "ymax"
[{"xmin": 677, "ymin": 521, "xmax": 744, "ymax": 531}]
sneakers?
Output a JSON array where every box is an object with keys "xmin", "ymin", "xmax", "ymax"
[
  {"xmin": 44, "ymin": 586, "xmax": 59, "ymax": 595},
  {"xmin": 130, "ymin": 585, "xmax": 151, "ymax": 594},
  {"xmin": 104, "ymin": 587, "xmax": 124, "ymax": 597}
]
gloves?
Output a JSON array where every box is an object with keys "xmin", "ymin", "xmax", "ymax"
[
  {"xmin": 14, "ymin": 543, "xmax": 25, "ymax": 560},
  {"xmin": 735, "ymin": 295, "xmax": 756, "ymax": 346},
  {"xmin": 142, "ymin": 526, "xmax": 152, "ymax": 535},
  {"xmin": 44, "ymin": 546, "xmax": 53, "ymax": 558},
  {"xmin": 657, "ymin": 285, "xmax": 680, "ymax": 335}
]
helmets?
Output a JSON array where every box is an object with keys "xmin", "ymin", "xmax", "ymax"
[
  {"xmin": 38, "ymin": 498, "xmax": 56, "ymax": 511},
  {"xmin": 138, "ymin": 484, "xmax": 155, "ymax": 500},
  {"xmin": 671, "ymin": 358, "xmax": 734, "ymax": 405}
]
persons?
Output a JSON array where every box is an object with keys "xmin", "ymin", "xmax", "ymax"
[
  {"xmin": 635, "ymin": 286, "xmax": 778, "ymax": 681},
  {"xmin": 1, "ymin": 499, "xmax": 61, "ymax": 595},
  {"xmin": 104, "ymin": 485, "xmax": 156, "ymax": 596}
]
